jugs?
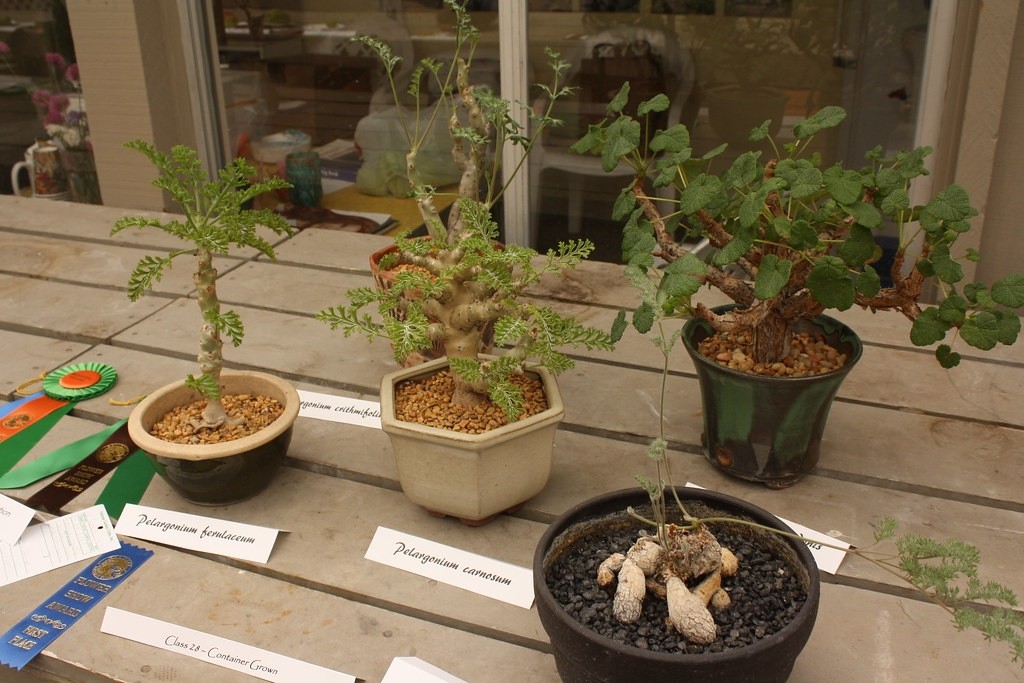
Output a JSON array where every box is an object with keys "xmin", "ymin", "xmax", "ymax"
[{"xmin": 10, "ymin": 137, "xmax": 71, "ymax": 204}]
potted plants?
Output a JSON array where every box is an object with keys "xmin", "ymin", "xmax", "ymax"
[
  {"xmin": 562, "ymin": 74, "xmax": 1023, "ymax": 492},
  {"xmin": 309, "ymin": 1, "xmax": 623, "ymax": 528},
  {"xmin": 108, "ymin": 134, "xmax": 301, "ymax": 505},
  {"xmin": 529, "ymin": 261, "xmax": 1024, "ymax": 682}
]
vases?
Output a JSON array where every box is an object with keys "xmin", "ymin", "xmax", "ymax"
[{"xmin": 61, "ymin": 142, "xmax": 102, "ymax": 206}]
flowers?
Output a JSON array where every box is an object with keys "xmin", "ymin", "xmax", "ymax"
[{"xmin": 35, "ymin": 50, "xmax": 89, "ymax": 154}]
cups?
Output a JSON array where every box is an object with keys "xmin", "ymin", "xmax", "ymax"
[{"xmin": 285, "ymin": 149, "xmax": 323, "ymax": 207}]
